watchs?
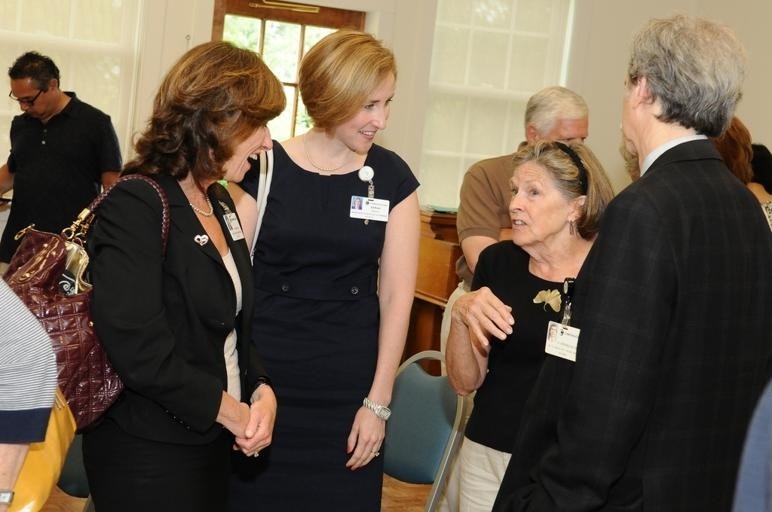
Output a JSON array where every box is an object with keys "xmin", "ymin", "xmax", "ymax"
[
  {"xmin": 0, "ymin": 489, "xmax": 14, "ymax": 506},
  {"xmin": 363, "ymin": 398, "xmax": 391, "ymax": 421}
]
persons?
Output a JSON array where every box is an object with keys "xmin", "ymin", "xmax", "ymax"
[
  {"xmin": 0, "ymin": 278, "xmax": 59, "ymax": 512},
  {"xmin": 492, "ymin": 15, "xmax": 772, "ymax": 510},
  {"xmin": 440, "ymin": 87, "xmax": 616, "ymax": 511},
  {"xmin": 621, "ymin": 117, "xmax": 770, "ymax": 512},
  {"xmin": 226, "ymin": 28, "xmax": 421, "ymax": 512},
  {"xmin": 0, "ymin": 51, "xmax": 122, "ymax": 278},
  {"xmin": 82, "ymin": 41, "xmax": 287, "ymax": 512}
]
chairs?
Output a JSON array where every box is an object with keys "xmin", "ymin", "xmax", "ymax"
[
  {"xmin": 732, "ymin": 379, "xmax": 772, "ymax": 512},
  {"xmin": 377, "ymin": 348, "xmax": 470, "ymax": 511}
]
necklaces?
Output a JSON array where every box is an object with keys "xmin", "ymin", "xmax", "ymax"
[
  {"xmin": 190, "ymin": 194, "xmax": 214, "ymax": 217},
  {"xmin": 303, "ymin": 134, "xmax": 345, "ymax": 172}
]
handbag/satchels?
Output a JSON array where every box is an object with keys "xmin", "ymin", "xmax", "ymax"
[{"xmin": 3, "ymin": 228, "xmax": 124, "ymax": 511}]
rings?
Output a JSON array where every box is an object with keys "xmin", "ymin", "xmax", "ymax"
[
  {"xmin": 371, "ymin": 450, "xmax": 380, "ymax": 457},
  {"xmin": 253, "ymin": 450, "xmax": 259, "ymax": 458}
]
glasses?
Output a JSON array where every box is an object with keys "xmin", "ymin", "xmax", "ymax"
[{"xmin": 9, "ymin": 90, "xmax": 42, "ymax": 106}]
